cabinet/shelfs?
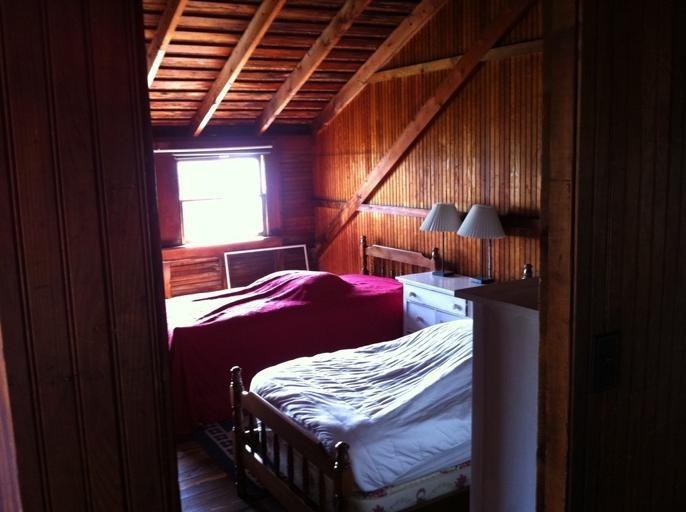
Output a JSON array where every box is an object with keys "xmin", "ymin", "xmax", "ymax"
[{"xmin": 454, "ymin": 276, "xmax": 537, "ymax": 512}]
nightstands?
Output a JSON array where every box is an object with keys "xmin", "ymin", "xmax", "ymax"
[{"xmin": 395, "ymin": 270, "xmax": 490, "ymax": 337}]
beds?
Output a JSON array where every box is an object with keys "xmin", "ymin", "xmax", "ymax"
[
  {"xmin": 163, "ymin": 235, "xmax": 440, "ymax": 434},
  {"xmin": 228, "ymin": 317, "xmax": 473, "ymax": 511}
]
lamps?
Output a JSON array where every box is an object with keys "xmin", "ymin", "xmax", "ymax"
[
  {"xmin": 418, "ymin": 203, "xmax": 463, "ymax": 277},
  {"xmin": 457, "ymin": 203, "xmax": 506, "ymax": 284}
]
